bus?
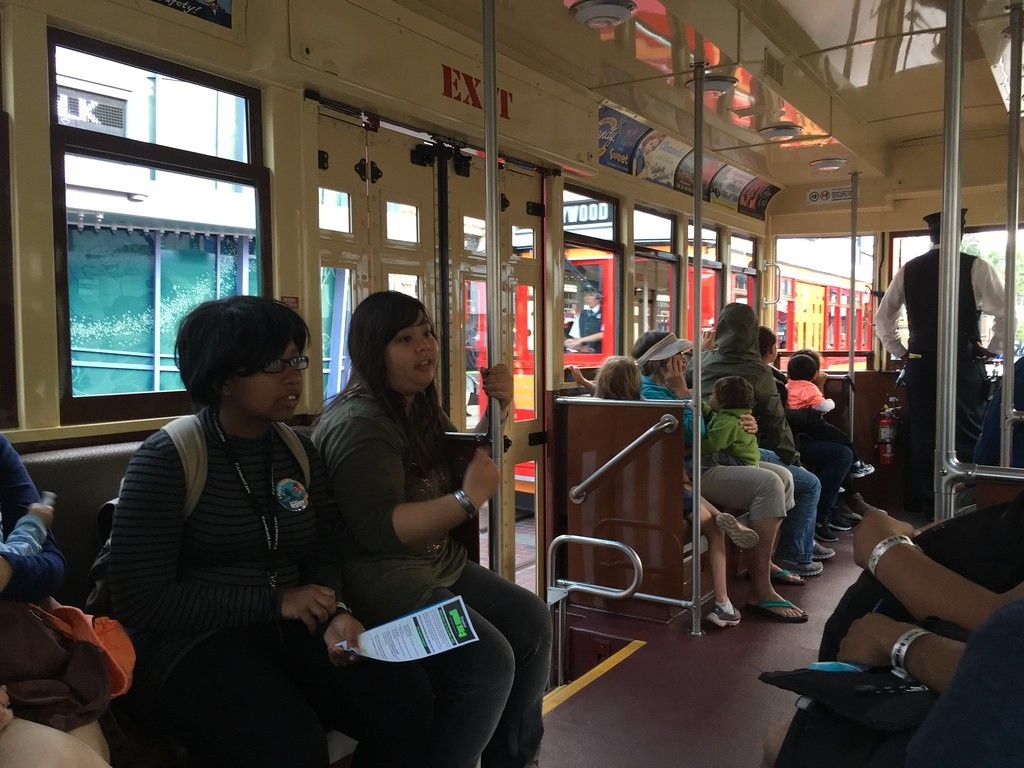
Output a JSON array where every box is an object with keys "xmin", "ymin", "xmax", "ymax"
[{"xmin": 466, "ymin": 194, "xmax": 874, "ymax": 513}]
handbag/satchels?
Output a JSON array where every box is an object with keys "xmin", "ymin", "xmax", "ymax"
[
  {"xmin": 758, "ymin": 615, "xmax": 973, "ymax": 734},
  {"xmin": 25, "ymin": 599, "xmax": 136, "ymax": 699}
]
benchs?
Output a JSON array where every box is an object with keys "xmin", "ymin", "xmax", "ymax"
[{"xmin": 16, "ymin": 425, "xmax": 314, "ymax": 615}]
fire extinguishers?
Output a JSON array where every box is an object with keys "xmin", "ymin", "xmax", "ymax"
[{"xmin": 870, "ymin": 404, "xmax": 901, "ymax": 467}]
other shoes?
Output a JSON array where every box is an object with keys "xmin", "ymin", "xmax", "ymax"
[
  {"xmin": 815, "ymin": 522, "xmax": 839, "ymax": 541},
  {"xmin": 716, "ymin": 513, "xmax": 759, "ymax": 550},
  {"xmin": 829, "ymin": 515, "xmax": 852, "ymax": 530}
]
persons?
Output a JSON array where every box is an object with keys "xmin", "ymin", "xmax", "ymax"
[
  {"xmin": 0, "ymin": 435, "xmax": 111, "ymax": 768},
  {"xmin": 310, "ymin": 288, "xmax": 551, "ymax": 768},
  {"xmin": 875, "ymin": 206, "xmax": 1019, "ymax": 521},
  {"xmin": 563, "ymin": 279, "xmax": 889, "ymax": 627},
  {"xmin": 81, "ymin": 291, "xmax": 438, "ymax": 768},
  {"xmin": 775, "ymin": 490, "xmax": 1024, "ymax": 768}
]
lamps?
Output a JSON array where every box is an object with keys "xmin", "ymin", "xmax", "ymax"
[
  {"xmin": 568, "ymin": 0, "xmax": 637, "ymax": 28},
  {"xmin": 684, "ymin": 76, "xmax": 738, "ymax": 97},
  {"xmin": 811, "ymin": 157, "xmax": 847, "ymax": 171},
  {"xmin": 757, "ymin": 126, "xmax": 803, "ymax": 143}
]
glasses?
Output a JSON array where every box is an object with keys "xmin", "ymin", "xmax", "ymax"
[{"xmin": 261, "ymin": 355, "xmax": 310, "ymax": 373}]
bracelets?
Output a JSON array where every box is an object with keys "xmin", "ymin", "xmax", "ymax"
[
  {"xmin": 336, "ymin": 601, "xmax": 352, "ymax": 613},
  {"xmin": 869, "ymin": 534, "xmax": 923, "ymax": 577},
  {"xmin": 891, "ymin": 628, "xmax": 932, "ymax": 683},
  {"xmin": 452, "ymin": 489, "xmax": 478, "ymax": 519},
  {"xmin": 483, "ymin": 407, "xmax": 509, "ymax": 426}
]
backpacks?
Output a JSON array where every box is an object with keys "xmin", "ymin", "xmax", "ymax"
[{"xmin": 85, "ymin": 415, "xmax": 311, "ymax": 615}]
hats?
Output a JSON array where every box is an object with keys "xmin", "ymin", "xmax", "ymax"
[
  {"xmin": 922, "ymin": 208, "xmax": 968, "ymax": 228},
  {"xmin": 636, "ymin": 333, "xmax": 693, "ymax": 369}
]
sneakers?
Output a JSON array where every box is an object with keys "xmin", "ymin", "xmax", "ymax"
[
  {"xmin": 706, "ymin": 604, "xmax": 742, "ymax": 628},
  {"xmin": 771, "ymin": 549, "xmax": 823, "ymax": 576},
  {"xmin": 850, "ymin": 460, "xmax": 875, "ymax": 478},
  {"xmin": 812, "ymin": 540, "xmax": 835, "ymax": 559}
]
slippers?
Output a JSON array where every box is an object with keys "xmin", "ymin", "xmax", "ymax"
[
  {"xmin": 744, "ymin": 599, "xmax": 809, "ymax": 622},
  {"xmin": 745, "ymin": 568, "xmax": 805, "ymax": 585}
]
